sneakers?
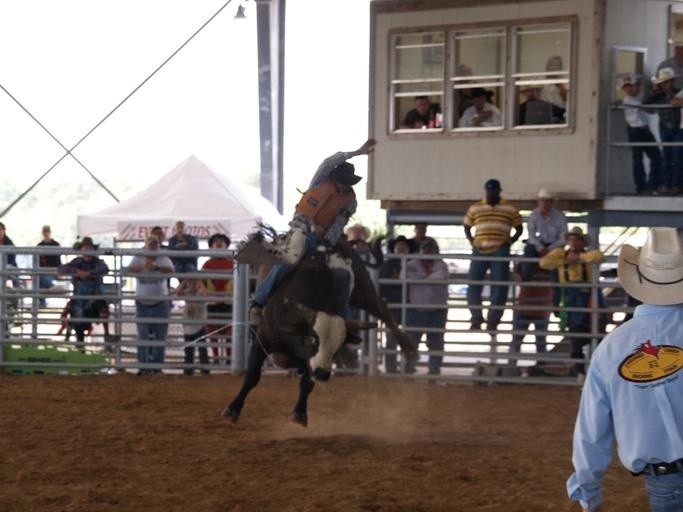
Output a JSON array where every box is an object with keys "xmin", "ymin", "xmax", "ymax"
[{"xmin": 250, "ymin": 307, "xmax": 262, "ymax": 321}]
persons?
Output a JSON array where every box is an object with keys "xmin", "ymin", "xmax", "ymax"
[
  {"xmin": 249, "ymin": 139, "xmax": 375, "ymax": 326},
  {"xmin": 512, "ymin": 187, "xmax": 564, "ymax": 315},
  {"xmin": 618, "ymin": 66, "xmax": 683, "ymax": 193},
  {"xmin": 57, "ymin": 237, "xmax": 110, "ymax": 353},
  {"xmin": 566, "ymin": 225, "xmax": 683, "ymax": 512},
  {"xmin": 462, "ymin": 179, "xmax": 524, "ymax": 335},
  {"xmin": 35, "ymin": 226, "xmax": 61, "ymax": 308},
  {"xmin": 0, "ymin": 222, "xmax": 17, "ymax": 273},
  {"xmin": 129, "ymin": 222, "xmax": 608, "ymax": 381},
  {"xmin": 394, "ymin": 57, "xmax": 570, "ymax": 127}
]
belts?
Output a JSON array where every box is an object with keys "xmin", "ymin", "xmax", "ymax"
[{"xmin": 631, "ymin": 459, "xmax": 682, "ymax": 478}]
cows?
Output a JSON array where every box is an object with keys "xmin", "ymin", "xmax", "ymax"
[{"xmin": 220, "ymin": 217, "xmax": 419, "ymax": 427}]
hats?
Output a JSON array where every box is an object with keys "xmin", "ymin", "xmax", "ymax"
[
  {"xmin": 620, "ymin": 73, "xmax": 637, "ymax": 88},
  {"xmin": 73, "ymin": 237, "xmax": 99, "ymax": 250},
  {"xmin": 652, "ymin": 67, "xmax": 680, "ymax": 86},
  {"xmin": 347, "ymin": 223, "xmax": 371, "ymax": 239},
  {"xmin": 617, "ymin": 227, "xmax": 683, "ymax": 305},
  {"xmin": 332, "ymin": 162, "xmax": 362, "ymax": 185},
  {"xmin": 485, "ymin": 180, "xmax": 500, "ymax": 188},
  {"xmin": 388, "ymin": 236, "xmax": 415, "ymax": 253},
  {"xmin": 207, "ymin": 233, "xmax": 230, "ymax": 247},
  {"xmin": 463, "ymin": 88, "xmax": 494, "ymax": 99},
  {"xmin": 561, "ymin": 226, "xmax": 592, "ymax": 247},
  {"xmin": 144, "ymin": 236, "xmax": 159, "ymax": 244},
  {"xmin": 538, "ymin": 188, "xmax": 559, "ymax": 202}
]
